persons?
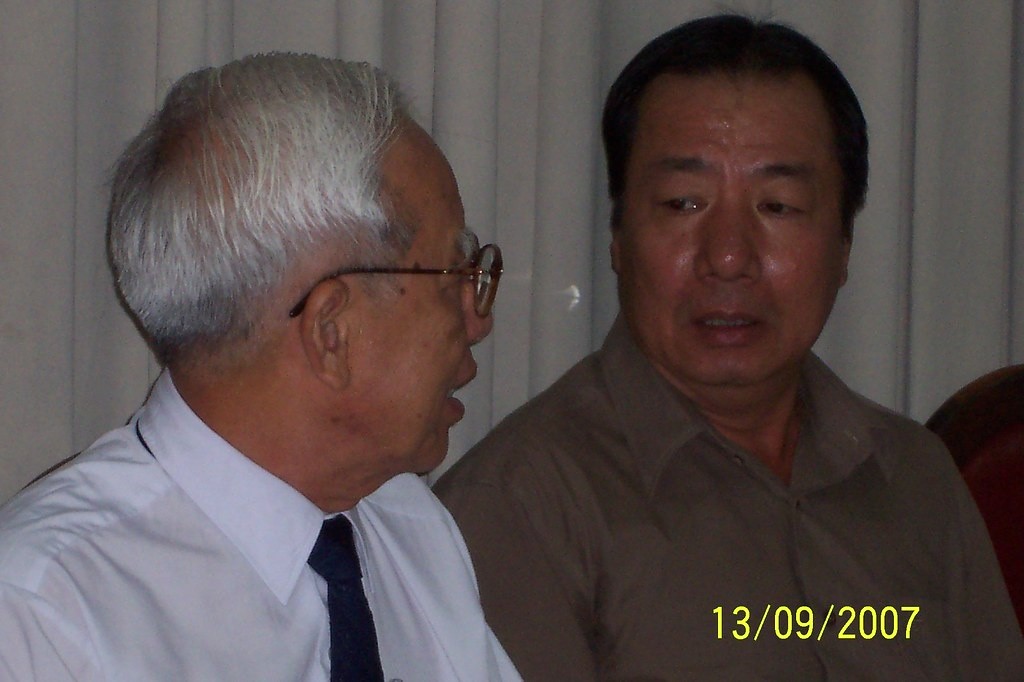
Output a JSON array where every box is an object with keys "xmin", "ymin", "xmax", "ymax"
[
  {"xmin": 0, "ymin": 49, "xmax": 525, "ymax": 682},
  {"xmin": 429, "ymin": 0, "xmax": 1024, "ymax": 682}
]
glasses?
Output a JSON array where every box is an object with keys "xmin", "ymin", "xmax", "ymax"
[{"xmin": 289, "ymin": 231, "xmax": 504, "ymax": 318}]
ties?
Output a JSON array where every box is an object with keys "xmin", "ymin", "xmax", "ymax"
[{"xmin": 307, "ymin": 513, "xmax": 384, "ymax": 682}]
